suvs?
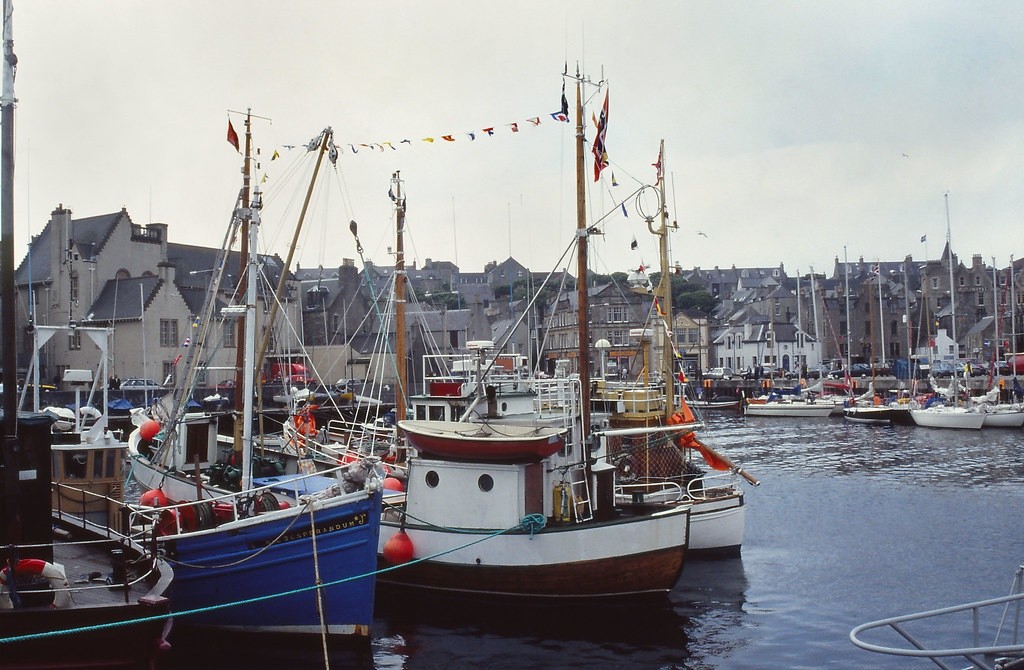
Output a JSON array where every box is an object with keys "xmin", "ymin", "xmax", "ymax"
[{"xmin": 702, "ymin": 366, "xmax": 734, "ymax": 380}]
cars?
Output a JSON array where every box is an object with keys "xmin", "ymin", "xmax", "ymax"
[
  {"xmin": 783, "ymin": 368, "xmax": 819, "ymax": 379},
  {"xmin": 118, "ymin": 378, "xmax": 166, "ymax": 390},
  {"xmin": 984, "ymin": 360, "xmax": 1012, "ymax": 377},
  {"xmin": 954, "ymin": 361, "xmax": 987, "ymax": 377},
  {"xmin": 739, "ymin": 366, "xmax": 775, "ymax": 381},
  {"xmin": 928, "ymin": 361, "xmax": 955, "ymax": 378},
  {"xmin": 828, "ymin": 363, "xmax": 873, "ymax": 380},
  {"xmin": 208, "ymin": 380, "xmax": 235, "ymax": 389},
  {"xmin": 873, "ymin": 363, "xmax": 891, "ymax": 378},
  {"xmin": 335, "ymin": 378, "xmax": 361, "ymax": 386}
]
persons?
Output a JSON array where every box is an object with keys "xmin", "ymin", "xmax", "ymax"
[
  {"xmin": 712, "ymin": 392, "xmax": 717, "ymax": 400},
  {"xmin": 747, "ymin": 363, "xmax": 764, "ymax": 379},
  {"xmin": 111, "ymin": 374, "xmax": 121, "ymax": 390},
  {"xmin": 706, "ymin": 366, "xmax": 714, "ymax": 373},
  {"xmin": 592, "ymin": 87, "xmax": 610, "ymax": 182},
  {"xmin": 735, "ymin": 384, "xmax": 741, "ymax": 401},
  {"xmin": 621, "ymin": 366, "xmax": 627, "ymax": 380},
  {"xmin": 964, "ymin": 359, "xmax": 972, "ymax": 380},
  {"xmin": 594, "ymin": 369, "xmax": 599, "ymax": 376}
]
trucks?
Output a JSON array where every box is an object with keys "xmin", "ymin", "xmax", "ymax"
[{"xmin": 253, "ymin": 363, "xmax": 319, "ymax": 386}]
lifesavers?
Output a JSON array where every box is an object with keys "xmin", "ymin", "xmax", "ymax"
[
  {"xmin": 0, "ymin": 559, "xmax": 70, "ymax": 608},
  {"xmin": 615, "ymin": 454, "xmax": 639, "ymax": 482}
]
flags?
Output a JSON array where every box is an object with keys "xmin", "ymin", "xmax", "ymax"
[
  {"xmin": 561, "ymin": 83, "xmax": 570, "ymax": 124},
  {"xmin": 921, "ymin": 235, "xmax": 926, "ymax": 242},
  {"xmin": 227, "ymin": 120, "xmax": 240, "ymax": 153}
]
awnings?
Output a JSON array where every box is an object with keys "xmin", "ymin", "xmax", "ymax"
[{"xmin": 271, "ymin": 362, "xmax": 309, "ymax": 379}]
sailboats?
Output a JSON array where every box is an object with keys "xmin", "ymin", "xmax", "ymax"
[{"xmin": 5, "ymin": 0, "xmax": 1024, "ymax": 670}]
